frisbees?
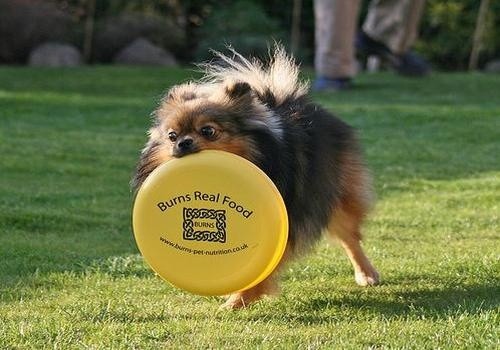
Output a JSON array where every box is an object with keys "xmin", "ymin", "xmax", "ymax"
[{"xmin": 132, "ymin": 149, "xmax": 289, "ymax": 297}]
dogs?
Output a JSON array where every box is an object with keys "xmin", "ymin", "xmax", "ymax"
[{"xmin": 129, "ymin": 36, "xmax": 381, "ymax": 312}]
